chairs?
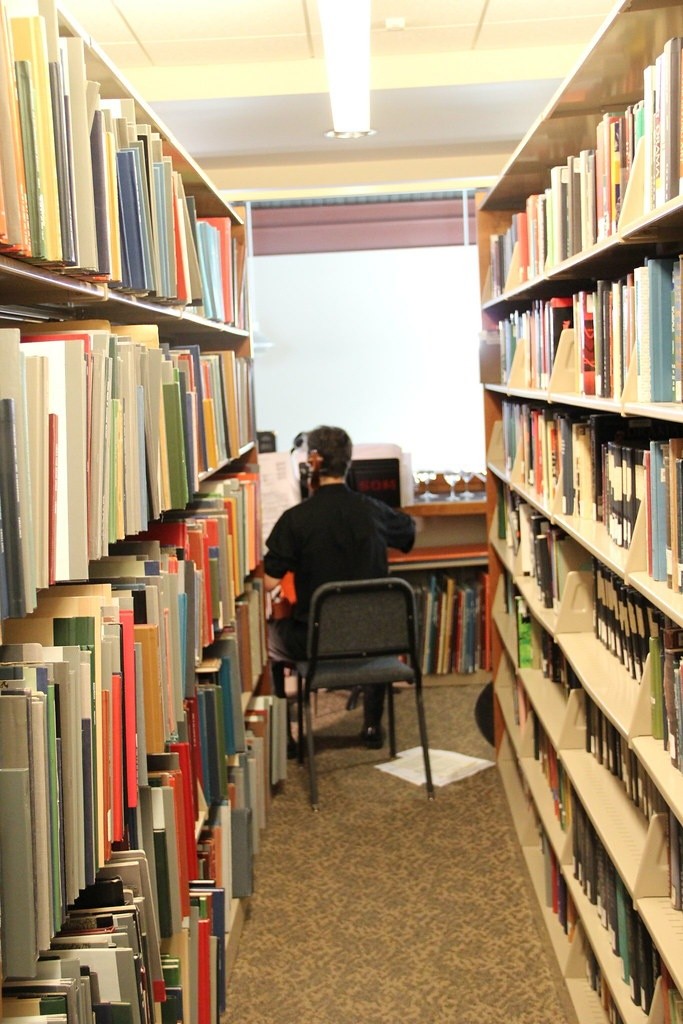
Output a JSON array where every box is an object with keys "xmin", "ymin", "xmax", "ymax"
[{"xmin": 293, "ymin": 578, "xmax": 436, "ymax": 812}]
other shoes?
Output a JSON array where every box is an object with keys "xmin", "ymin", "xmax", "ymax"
[
  {"xmin": 360, "ymin": 724, "xmax": 386, "ymax": 749},
  {"xmin": 286, "ymin": 736, "xmax": 309, "ymax": 759}
]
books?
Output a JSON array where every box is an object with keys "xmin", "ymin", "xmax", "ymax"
[
  {"xmin": 0, "ymin": 0, "xmax": 251, "ymax": 329},
  {"xmin": 496, "ymin": 249, "xmax": 683, "ymax": 409},
  {"xmin": 0, "ymin": 311, "xmax": 257, "ymax": 628},
  {"xmin": 494, "ymin": 474, "xmax": 683, "ymax": 1024},
  {"xmin": 0, "ymin": 460, "xmax": 291, "ymax": 1024},
  {"xmin": 401, "ymin": 568, "xmax": 494, "ymax": 677},
  {"xmin": 484, "ymin": 32, "xmax": 683, "ymax": 303},
  {"xmin": 497, "ymin": 399, "xmax": 683, "ymax": 601}
]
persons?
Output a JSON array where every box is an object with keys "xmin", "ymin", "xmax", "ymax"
[{"xmin": 263, "ymin": 424, "xmax": 417, "ymax": 760}]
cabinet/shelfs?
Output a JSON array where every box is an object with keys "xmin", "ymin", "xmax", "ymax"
[
  {"xmin": 0, "ymin": 0, "xmax": 274, "ymax": 1024},
  {"xmin": 388, "ymin": 502, "xmax": 486, "ymax": 687},
  {"xmin": 472, "ymin": 0, "xmax": 683, "ymax": 1024}
]
